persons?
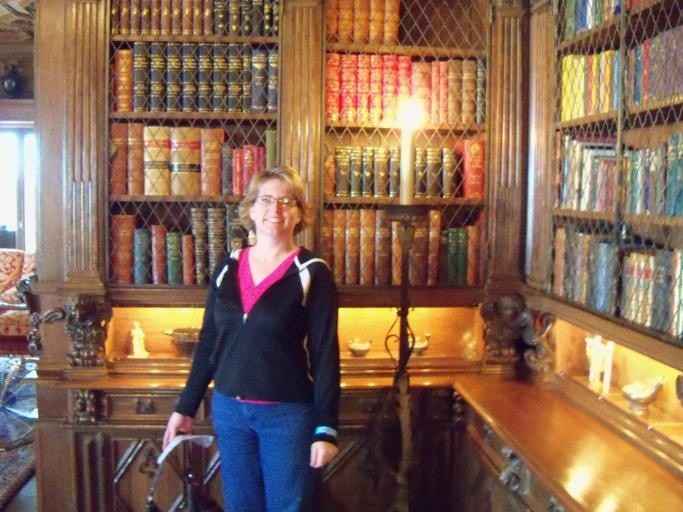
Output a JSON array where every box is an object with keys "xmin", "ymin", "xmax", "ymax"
[
  {"xmin": 130, "ymin": 322, "xmax": 147, "ymax": 355},
  {"xmin": 162, "ymin": 163, "xmax": 340, "ymax": 512}
]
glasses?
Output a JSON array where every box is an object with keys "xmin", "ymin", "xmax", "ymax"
[{"xmin": 257, "ymin": 196, "xmax": 298, "ymax": 208}]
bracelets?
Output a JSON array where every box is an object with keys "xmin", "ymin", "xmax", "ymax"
[{"xmin": 314, "ymin": 424, "xmax": 336, "ymax": 440}]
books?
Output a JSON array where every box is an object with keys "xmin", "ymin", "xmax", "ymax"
[
  {"xmin": 108, "ymin": 122, "xmax": 277, "ymax": 196},
  {"xmin": 326, "ymin": 52, "xmax": 486, "ymax": 124},
  {"xmin": 554, "ymin": 1, "xmax": 683, "ymax": 343},
  {"xmin": 322, "ymin": 208, "xmax": 479, "ymax": 286},
  {"xmin": 328, "ymin": 0, "xmax": 400, "ymax": 43},
  {"xmin": 324, "ymin": 145, "xmax": 400, "ymax": 198},
  {"xmin": 110, "ymin": 1, "xmax": 279, "ymax": 38},
  {"xmin": 109, "ymin": 42, "xmax": 278, "ymax": 113},
  {"xmin": 109, "ymin": 203, "xmax": 256, "ymax": 286},
  {"xmin": 414, "ymin": 139, "xmax": 484, "ymax": 199}
]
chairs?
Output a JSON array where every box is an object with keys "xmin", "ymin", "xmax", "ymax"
[
  {"xmin": 0, "ymin": 249, "xmax": 37, "ymax": 342},
  {"xmin": 0, "ymin": 248, "xmax": 24, "ymax": 292}
]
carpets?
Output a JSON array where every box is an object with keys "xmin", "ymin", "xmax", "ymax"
[{"xmin": 0, "ymin": 353, "xmax": 37, "ymax": 512}]
